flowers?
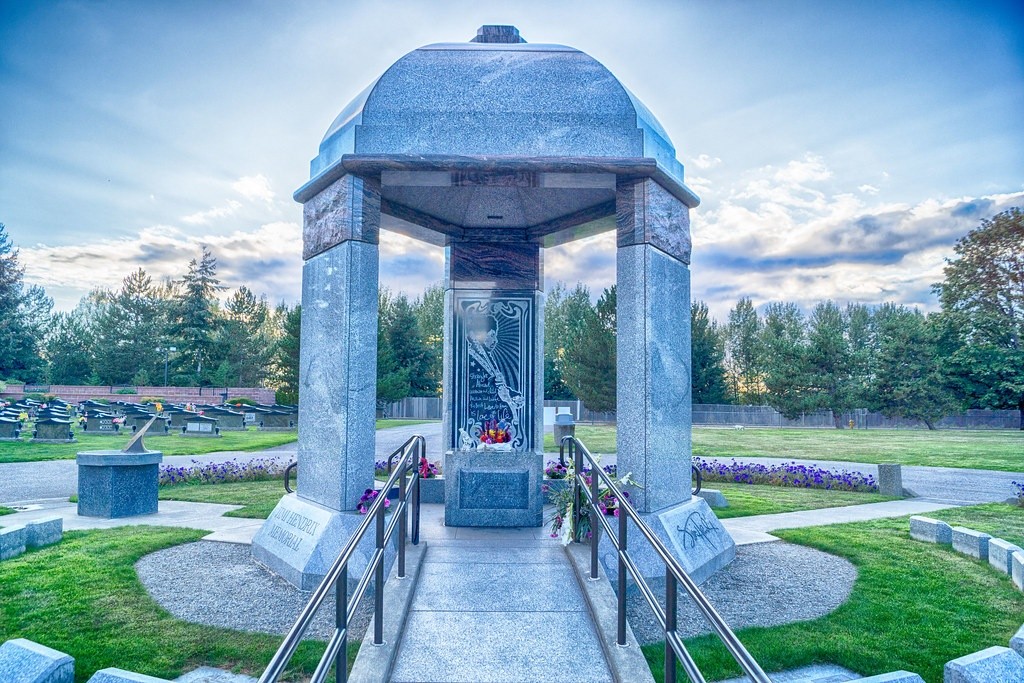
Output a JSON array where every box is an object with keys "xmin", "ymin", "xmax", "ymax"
[
  {"xmin": 418, "ymin": 458, "xmax": 438, "ymax": 478},
  {"xmin": 356, "ymin": 489, "xmax": 391, "ymax": 515},
  {"xmin": 480, "ymin": 420, "xmax": 512, "ymax": 444},
  {"xmin": 541, "ymin": 452, "xmax": 645, "ymax": 542}
]
